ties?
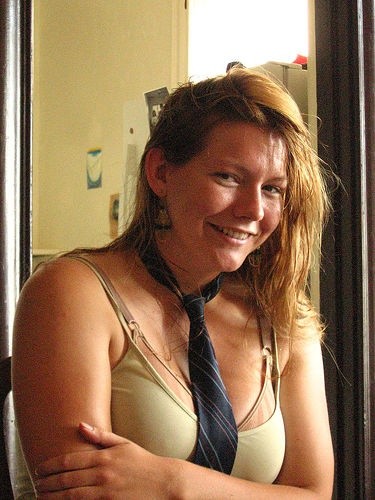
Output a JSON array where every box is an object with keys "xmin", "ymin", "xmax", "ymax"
[{"xmin": 134, "ymin": 228, "xmax": 239, "ymax": 475}]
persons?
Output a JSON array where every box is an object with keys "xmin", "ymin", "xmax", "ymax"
[{"xmin": 10, "ymin": 65, "xmax": 336, "ymax": 500}]
